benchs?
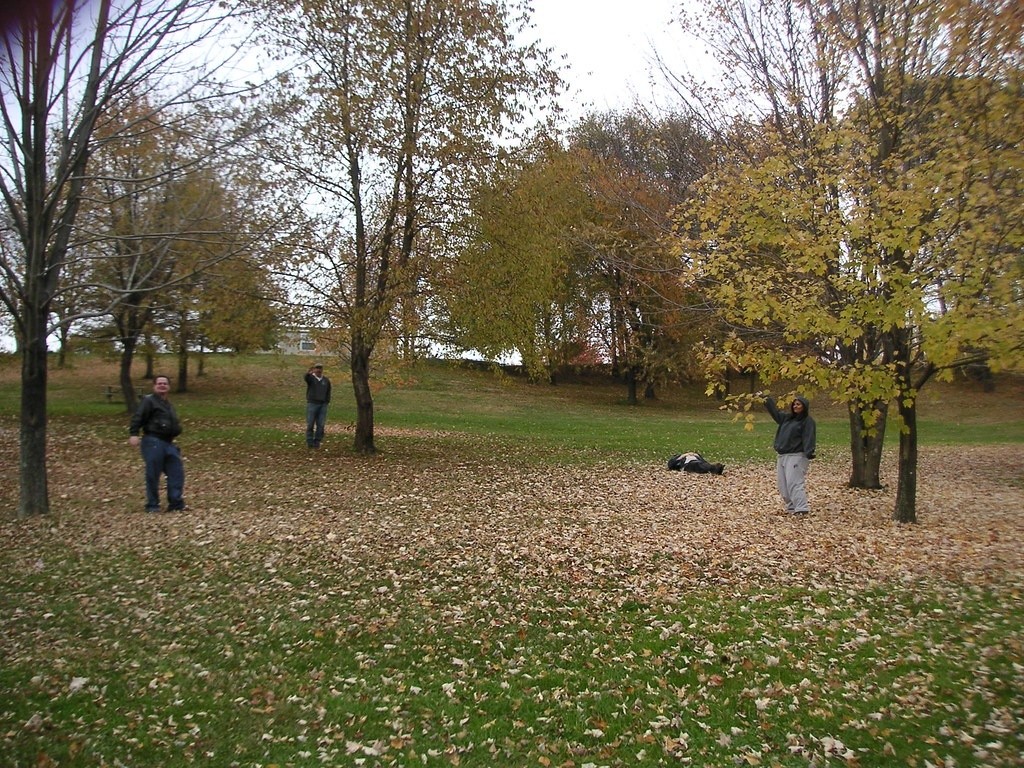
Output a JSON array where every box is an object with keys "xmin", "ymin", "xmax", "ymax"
[{"xmin": 101, "ymin": 385, "xmax": 146, "ymax": 404}]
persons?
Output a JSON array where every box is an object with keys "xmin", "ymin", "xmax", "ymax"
[
  {"xmin": 667, "ymin": 451, "xmax": 726, "ymax": 475},
  {"xmin": 755, "ymin": 391, "xmax": 816, "ymax": 516},
  {"xmin": 304, "ymin": 363, "xmax": 332, "ymax": 450},
  {"xmin": 129, "ymin": 375, "xmax": 189, "ymax": 513}
]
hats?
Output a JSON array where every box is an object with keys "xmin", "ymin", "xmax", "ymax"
[{"xmin": 315, "ymin": 363, "xmax": 323, "ymax": 368}]
